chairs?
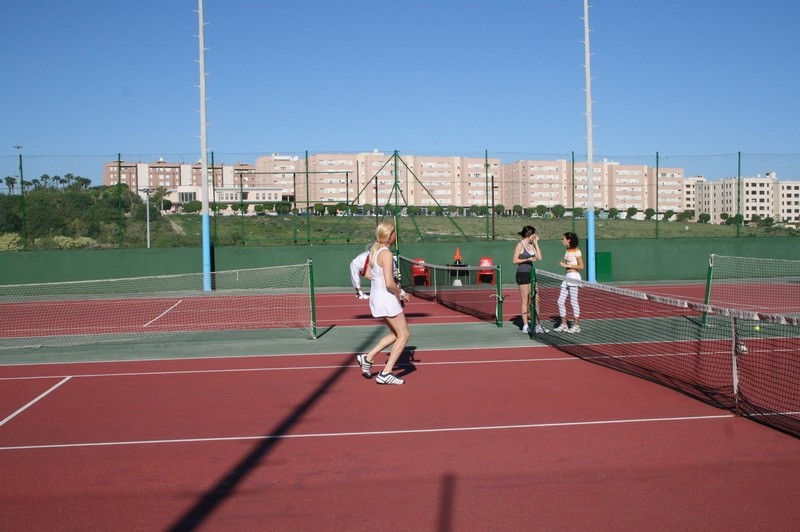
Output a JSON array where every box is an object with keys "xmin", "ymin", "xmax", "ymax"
[
  {"xmin": 476, "ymin": 256, "xmax": 496, "ymax": 285},
  {"xmin": 412, "ymin": 258, "xmax": 431, "ymax": 286}
]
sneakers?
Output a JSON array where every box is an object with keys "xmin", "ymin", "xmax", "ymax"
[
  {"xmin": 553, "ymin": 324, "xmax": 569, "ymax": 333},
  {"xmin": 357, "ymin": 354, "xmax": 374, "ymax": 378},
  {"xmin": 567, "ymin": 325, "xmax": 580, "ymax": 333},
  {"xmin": 522, "ymin": 325, "xmax": 531, "ymax": 333},
  {"xmin": 375, "ymin": 371, "xmax": 405, "ymax": 385},
  {"xmin": 535, "ymin": 325, "xmax": 550, "ymax": 334}
]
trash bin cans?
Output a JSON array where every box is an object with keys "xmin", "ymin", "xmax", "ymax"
[{"xmin": 596, "ymin": 252, "xmax": 613, "ymax": 282}]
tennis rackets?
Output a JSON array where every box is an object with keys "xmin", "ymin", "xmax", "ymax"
[{"xmin": 392, "ymin": 257, "xmax": 406, "ymax": 308}]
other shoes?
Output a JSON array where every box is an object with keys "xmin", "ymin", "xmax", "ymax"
[
  {"xmin": 356, "ymin": 293, "xmax": 360, "ymax": 298},
  {"xmin": 358, "ymin": 294, "xmax": 370, "ymax": 299}
]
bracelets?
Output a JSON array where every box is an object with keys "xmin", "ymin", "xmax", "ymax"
[
  {"xmin": 527, "ymin": 258, "xmax": 529, "ymax": 262},
  {"xmin": 399, "ymin": 289, "xmax": 406, "ymax": 298}
]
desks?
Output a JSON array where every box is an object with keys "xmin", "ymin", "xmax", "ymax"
[{"xmin": 445, "ymin": 264, "xmax": 471, "ymax": 286}]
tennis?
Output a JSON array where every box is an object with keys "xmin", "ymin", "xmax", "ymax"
[{"xmin": 755, "ymin": 326, "xmax": 760, "ymax": 331}]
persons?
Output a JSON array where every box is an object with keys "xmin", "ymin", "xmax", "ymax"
[
  {"xmin": 356, "ymin": 222, "xmax": 411, "ymax": 385},
  {"xmin": 512, "ymin": 226, "xmax": 551, "ymax": 334},
  {"xmin": 349, "ymin": 251, "xmax": 373, "ymax": 300},
  {"xmin": 552, "ymin": 232, "xmax": 584, "ymax": 334}
]
